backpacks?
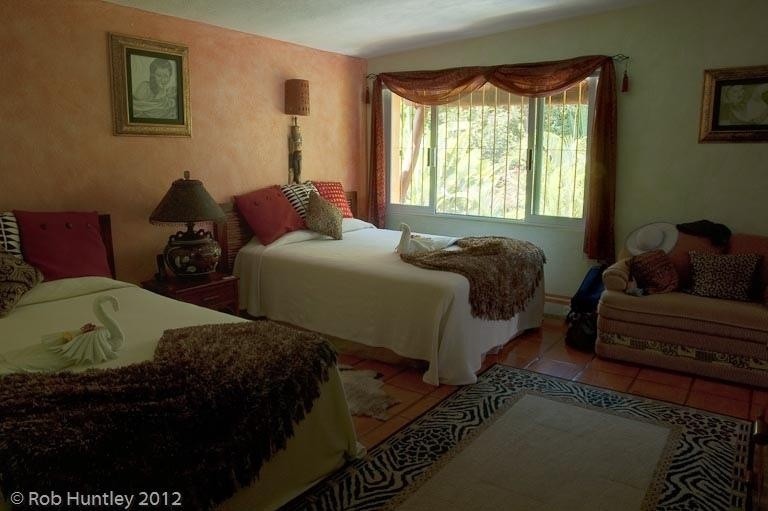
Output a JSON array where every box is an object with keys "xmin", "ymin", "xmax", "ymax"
[{"xmin": 571, "ymin": 262, "xmax": 610, "ymax": 313}]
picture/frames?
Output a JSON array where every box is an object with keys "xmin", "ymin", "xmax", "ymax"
[
  {"xmin": 109, "ymin": 28, "xmax": 196, "ymax": 143},
  {"xmin": 697, "ymin": 61, "xmax": 768, "ymax": 148}
]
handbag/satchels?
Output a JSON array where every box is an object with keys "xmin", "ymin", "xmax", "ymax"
[{"xmin": 629, "ymin": 249, "xmax": 680, "ymax": 294}]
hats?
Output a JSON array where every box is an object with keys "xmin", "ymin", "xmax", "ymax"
[{"xmin": 624, "ymin": 222, "xmax": 679, "ymax": 256}]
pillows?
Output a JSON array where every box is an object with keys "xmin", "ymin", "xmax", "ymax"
[
  {"xmin": 280, "ymin": 178, "xmax": 356, "ymax": 241},
  {"xmin": 1, "ymin": 204, "xmax": 117, "ymax": 317},
  {"xmin": 622, "ymin": 245, "xmax": 679, "ymax": 297},
  {"xmin": 232, "ymin": 182, "xmax": 308, "ymax": 246},
  {"xmin": 687, "ymin": 244, "xmax": 763, "ymax": 303}
]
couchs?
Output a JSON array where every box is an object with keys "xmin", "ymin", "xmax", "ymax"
[{"xmin": 595, "ymin": 212, "xmax": 768, "ymax": 388}]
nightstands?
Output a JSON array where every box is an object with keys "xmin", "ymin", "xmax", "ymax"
[{"xmin": 140, "ymin": 275, "xmax": 240, "ymax": 319}]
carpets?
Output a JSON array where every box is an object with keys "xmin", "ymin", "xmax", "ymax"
[{"xmin": 272, "ymin": 358, "xmax": 761, "ymax": 511}]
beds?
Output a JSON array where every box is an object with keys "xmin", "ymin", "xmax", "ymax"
[
  {"xmin": 212, "ymin": 191, "xmax": 553, "ymax": 385},
  {"xmin": 1, "ymin": 200, "xmax": 375, "ymax": 510}
]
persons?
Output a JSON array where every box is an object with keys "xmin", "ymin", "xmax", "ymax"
[
  {"xmin": 715, "ymin": 84, "xmax": 756, "ymax": 126},
  {"xmin": 131, "ymin": 54, "xmax": 181, "ymax": 120}
]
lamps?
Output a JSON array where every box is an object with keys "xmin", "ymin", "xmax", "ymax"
[
  {"xmin": 144, "ymin": 171, "xmax": 230, "ymax": 277},
  {"xmin": 283, "ymin": 79, "xmax": 311, "ymax": 184}
]
